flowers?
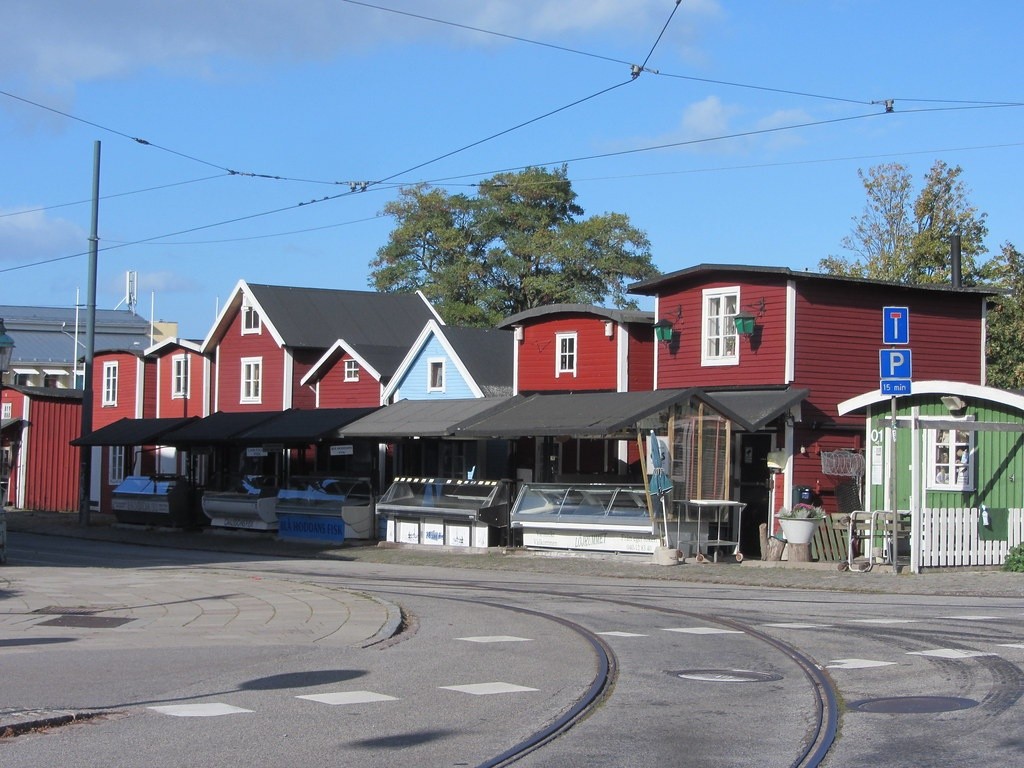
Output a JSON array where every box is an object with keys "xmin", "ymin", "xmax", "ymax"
[{"xmin": 778, "ymin": 502, "xmax": 826, "ymax": 518}]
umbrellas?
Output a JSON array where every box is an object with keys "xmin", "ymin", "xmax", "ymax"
[{"xmin": 649, "ymin": 431, "xmax": 673, "ymax": 547}]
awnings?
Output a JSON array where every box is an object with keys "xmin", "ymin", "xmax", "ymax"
[
  {"xmin": 243, "ymin": 404, "xmax": 387, "ymax": 444},
  {"xmin": 68, "ymin": 415, "xmax": 200, "ymax": 446},
  {"xmin": 449, "ymin": 389, "xmax": 809, "ymax": 439},
  {"xmin": 160, "ymin": 408, "xmax": 291, "ymax": 444},
  {"xmin": 335, "ymin": 394, "xmax": 524, "ymax": 439},
  {"xmin": 1, "ymin": 419, "xmax": 22, "ymax": 430}
]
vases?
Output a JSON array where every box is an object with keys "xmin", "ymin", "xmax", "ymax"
[{"xmin": 776, "ymin": 516, "xmax": 827, "ymax": 543}]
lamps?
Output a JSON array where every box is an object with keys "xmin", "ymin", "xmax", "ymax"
[
  {"xmin": 940, "ymin": 395, "xmax": 968, "ymax": 411},
  {"xmin": 511, "ymin": 325, "xmax": 523, "ymax": 341},
  {"xmin": 599, "ymin": 319, "xmax": 613, "ymax": 337},
  {"xmin": 652, "ymin": 304, "xmax": 682, "ymax": 348},
  {"xmin": 733, "ymin": 298, "xmax": 765, "ymax": 343}
]
